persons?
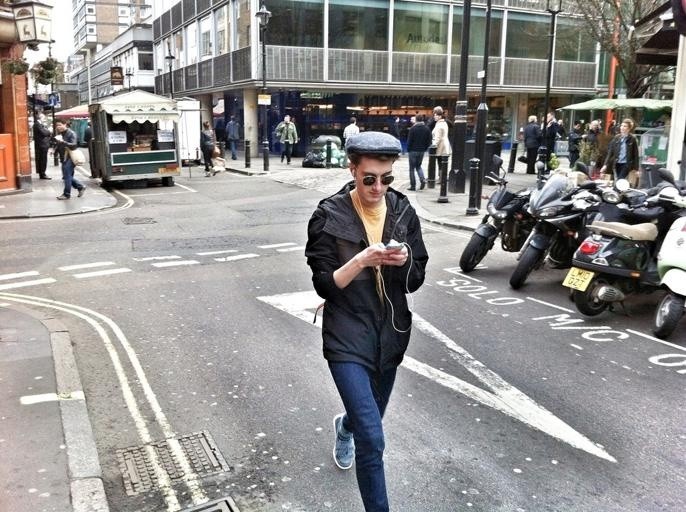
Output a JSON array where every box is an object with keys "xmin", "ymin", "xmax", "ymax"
[
  {"xmin": 388, "ymin": 104, "xmax": 639, "ymax": 190},
  {"xmin": 275, "ymin": 114, "xmax": 298, "ymax": 166},
  {"xmin": 201, "ymin": 121, "xmax": 221, "ymax": 177},
  {"xmin": 84, "ymin": 123, "xmax": 92, "ymax": 142},
  {"xmin": 304, "ymin": 131, "xmax": 431, "ymax": 512},
  {"xmin": 225, "ymin": 116, "xmax": 241, "ymax": 162},
  {"xmin": 341, "ymin": 117, "xmax": 360, "ymax": 169},
  {"xmin": 32, "ymin": 112, "xmax": 55, "ymax": 181},
  {"xmin": 51, "ymin": 120, "xmax": 86, "ymax": 200}
]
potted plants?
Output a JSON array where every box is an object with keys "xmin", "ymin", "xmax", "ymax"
[
  {"xmin": 31, "ymin": 57, "xmax": 64, "ymax": 86},
  {"xmin": 0, "ymin": 56, "xmax": 29, "ymax": 75}
]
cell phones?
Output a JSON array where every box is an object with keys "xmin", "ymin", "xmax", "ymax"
[{"xmin": 385, "ymin": 243, "xmax": 404, "ymax": 251}]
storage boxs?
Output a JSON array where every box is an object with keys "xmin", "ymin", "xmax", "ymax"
[
  {"xmin": 154, "ymin": 130, "xmax": 174, "ymax": 143},
  {"xmin": 109, "ymin": 144, "xmax": 127, "ymax": 152},
  {"xmin": 153, "ymin": 140, "xmax": 175, "ymax": 150},
  {"xmin": 156, "ymin": 119, "xmax": 174, "ymax": 130},
  {"xmin": 108, "ymin": 131, "xmax": 127, "ymax": 144}
]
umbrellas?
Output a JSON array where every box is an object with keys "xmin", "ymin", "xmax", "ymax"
[{"xmin": 48, "ymin": 103, "xmax": 93, "ymax": 119}]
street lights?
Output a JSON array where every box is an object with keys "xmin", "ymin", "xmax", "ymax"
[
  {"xmin": 164, "ymin": 41, "xmax": 174, "ymax": 98},
  {"xmin": 255, "ymin": 5, "xmax": 272, "ymax": 172},
  {"xmin": 125, "ymin": 64, "xmax": 134, "ymax": 91},
  {"xmin": 538, "ymin": 0, "xmax": 564, "ymax": 190},
  {"xmin": 78, "ymin": 48, "xmax": 92, "ymax": 107}
]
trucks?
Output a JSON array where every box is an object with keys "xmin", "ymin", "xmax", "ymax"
[{"xmin": 89, "ymin": 102, "xmax": 181, "ymax": 190}]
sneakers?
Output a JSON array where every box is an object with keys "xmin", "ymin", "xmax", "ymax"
[
  {"xmin": 407, "ymin": 186, "xmax": 415, "ymax": 191},
  {"xmin": 77, "ymin": 185, "xmax": 86, "ymax": 197},
  {"xmin": 56, "ymin": 193, "xmax": 70, "ymax": 200},
  {"xmin": 331, "ymin": 413, "xmax": 355, "ymax": 470},
  {"xmin": 287, "ymin": 158, "xmax": 292, "ymax": 164},
  {"xmin": 420, "ymin": 181, "xmax": 425, "ymax": 190},
  {"xmin": 212, "ymin": 169, "xmax": 217, "ymax": 176},
  {"xmin": 203, "ymin": 172, "xmax": 210, "ymax": 177}
]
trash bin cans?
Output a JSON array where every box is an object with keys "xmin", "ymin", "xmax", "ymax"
[
  {"xmin": 640, "ymin": 162, "xmax": 666, "ymax": 188},
  {"xmin": 464, "ymin": 139, "xmax": 501, "ymax": 186}
]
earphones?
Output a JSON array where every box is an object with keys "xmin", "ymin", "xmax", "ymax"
[{"xmin": 352, "ymin": 169, "xmax": 356, "ymax": 177}]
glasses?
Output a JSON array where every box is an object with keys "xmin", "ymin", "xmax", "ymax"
[{"xmin": 357, "ymin": 169, "xmax": 395, "ymax": 186}]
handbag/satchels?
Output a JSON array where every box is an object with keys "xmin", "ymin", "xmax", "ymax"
[
  {"xmin": 68, "ymin": 148, "xmax": 87, "ymax": 166},
  {"xmin": 274, "ymin": 122, "xmax": 284, "ymax": 137},
  {"xmin": 211, "ymin": 145, "xmax": 221, "ymax": 159},
  {"xmin": 517, "ymin": 148, "xmax": 528, "ymax": 164}
]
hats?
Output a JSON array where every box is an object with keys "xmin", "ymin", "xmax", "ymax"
[{"xmin": 345, "ymin": 130, "xmax": 403, "ymax": 164}]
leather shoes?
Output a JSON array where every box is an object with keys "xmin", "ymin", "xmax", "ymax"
[{"xmin": 40, "ymin": 175, "xmax": 52, "ymax": 180}]
